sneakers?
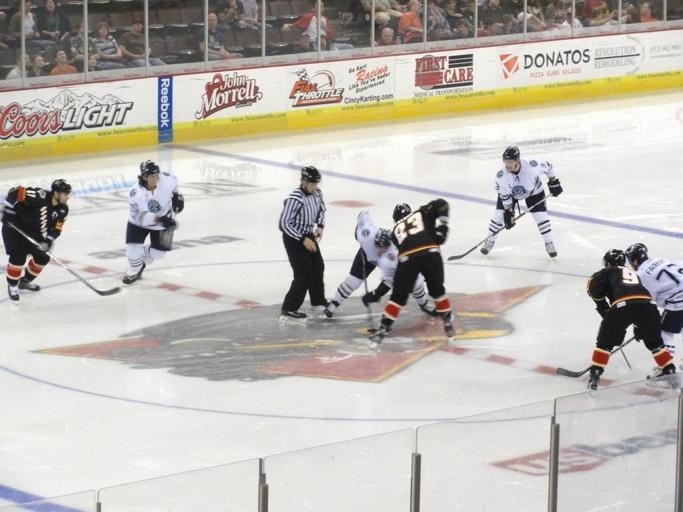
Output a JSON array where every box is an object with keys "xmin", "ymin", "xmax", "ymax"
[{"xmin": 282, "ymin": 310, "xmax": 306, "ymax": 318}]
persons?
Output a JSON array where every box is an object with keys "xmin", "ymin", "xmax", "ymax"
[
  {"xmin": 480, "ymin": 145, "xmax": 563, "ymax": 258},
  {"xmin": 626, "ymin": 243, "xmax": 683, "ymax": 359},
  {"xmin": 196, "ymin": 0, "xmax": 273, "ymax": 60},
  {"xmin": 122, "ymin": 159, "xmax": 185, "ymax": 284},
  {"xmin": 346, "ymin": 1, "xmax": 683, "ymax": 48},
  {"xmin": 369, "ymin": 198, "xmax": 456, "ymax": 341},
  {"xmin": 277, "ymin": 165, "xmax": 331, "ymax": 319},
  {"xmin": 279, "ymin": 1, "xmax": 336, "ymax": 52},
  {"xmin": 319, "ymin": 210, "xmax": 455, "ymax": 324},
  {"xmin": 1, "ymin": 179, "xmax": 72, "ymax": 300},
  {"xmin": 0, "ymin": 0, "xmax": 167, "ymax": 81},
  {"xmin": 586, "ymin": 248, "xmax": 675, "ymax": 390}
]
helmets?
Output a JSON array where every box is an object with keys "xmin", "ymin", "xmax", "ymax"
[
  {"xmin": 52, "ymin": 179, "xmax": 72, "ymax": 192},
  {"xmin": 393, "ymin": 203, "xmax": 412, "ymax": 222},
  {"xmin": 301, "ymin": 165, "xmax": 321, "ymax": 182},
  {"xmin": 626, "ymin": 242, "xmax": 649, "ymax": 262},
  {"xmin": 375, "ymin": 228, "xmax": 392, "ymax": 247},
  {"xmin": 603, "ymin": 249, "xmax": 626, "ymax": 267},
  {"xmin": 502, "ymin": 147, "xmax": 520, "ymax": 160},
  {"xmin": 140, "ymin": 159, "xmax": 160, "ymax": 176}
]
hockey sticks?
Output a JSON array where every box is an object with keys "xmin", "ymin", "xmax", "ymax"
[
  {"xmin": 7, "ymin": 221, "xmax": 119, "ymax": 295},
  {"xmin": 361, "ymin": 247, "xmax": 386, "ymax": 338},
  {"xmin": 448, "ymin": 194, "xmax": 553, "ymax": 260},
  {"xmin": 556, "ymin": 336, "xmax": 635, "ymax": 377}
]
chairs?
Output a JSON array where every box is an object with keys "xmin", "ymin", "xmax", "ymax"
[{"xmin": 0, "ymin": 0, "xmax": 364, "ymax": 80}]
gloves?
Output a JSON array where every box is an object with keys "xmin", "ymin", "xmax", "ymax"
[
  {"xmin": 159, "ymin": 215, "xmax": 175, "ymax": 229},
  {"xmin": 36, "ymin": 238, "xmax": 53, "ymax": 253},
  {"xmin": 436, "ymin": 225, "xmax": 448, "ymax": 245},
  {"xmin": 363, "ymin": 288, "xmax": 385, "ymax": 307},
  {"xmin": 172, "ymin": 193, "xmax": 185, "ymax": 212},
  {"xmin": 1, "ymin": 205, "xmax": 16, "ymax": 227},
  {"xmin": 503, "ymin": 210, "xmax": 515, "ymax": 230},
  {"xmin": 547, "ymin": 177, "xmax": 562, "ymax": 197}
]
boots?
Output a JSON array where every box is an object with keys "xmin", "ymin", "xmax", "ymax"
[
  {"xmin": 7, "ymin": 282, "xmax": 20, "ymax": 301},
  {"xmin": 324, "ymin": 302, "xmax": 339, "ymax": 318},
  {"xmin": 124, "ymin": 262, "xmax": 146, "ymax": 284},
  {"xmin": 368, "ymin": 327, "xmax": 389, "ymax": 344},
  {"xmin": 662, "ymin": 364, "xmax": 680, "ymax": 387},
  {"xmin": 480, "ymin": 240, "xmax": 496, "ymax": 254},
  {"xmin": 546, "ymin": 241, "xmax": 557, "ymax": 256},
  {"xmin": 19, "ymin": 277, "xmax": 41, "ymax": 291},
  {"xmin": 586, "ymin": 367, "xmax": 604, "ymax": 390},
  {"xmin": 420, "ymin": 300, "xmax": 454, "ymax": 338}
]
